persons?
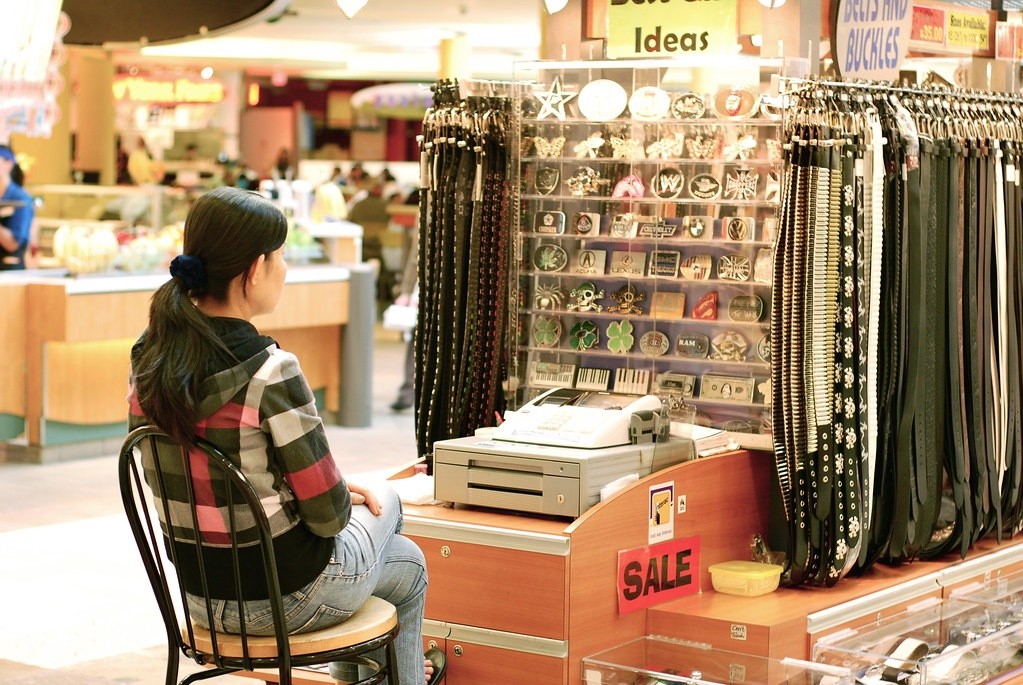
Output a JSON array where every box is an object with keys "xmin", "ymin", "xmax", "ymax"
[
  {"xmin": 0, "ymin": 143, "xmax": 33, "ymax": 271},
  {"xmin": 127, "ymin": 186, "xmax": 444, "ymax": 685}
]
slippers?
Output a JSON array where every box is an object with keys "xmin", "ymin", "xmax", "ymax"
[{"xmin": 425, "ymin": 647, "xmax": 447, "ymax": 685}]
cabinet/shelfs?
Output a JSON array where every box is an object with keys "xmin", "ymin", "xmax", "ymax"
[
  {"xmin": 804, "ymin": 542, "xmax": 1023, "ymax": 685},
  {"xmin": 400, "ymin": 515, "xmax": 570, "ymax": 684}
]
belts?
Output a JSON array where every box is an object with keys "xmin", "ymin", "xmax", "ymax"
[
  {"xmin": 857, "ymin": 617, "xmax": 1023, "ymax": 685},
  {"xmin": 412, "ymin": 94, "xmax": 524, "ymax": 477},
  {"xmin": 766, "ymin": 82, "xmax": 1023, "ymax": 588}
]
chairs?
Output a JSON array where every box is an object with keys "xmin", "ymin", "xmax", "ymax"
[{"xmin": 118, "ymin": 425, "xmax": 400, "ymax": 685}]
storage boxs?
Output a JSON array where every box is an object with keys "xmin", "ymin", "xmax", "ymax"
[
  {"xmin": 903, "ymin": 56, "xmax": 1007, "ymax": 93},
  {"xmin": 708, "ymin": 560, "xmax": 784, "ymax": 597}
]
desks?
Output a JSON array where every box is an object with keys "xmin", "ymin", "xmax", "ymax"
[
  {"xmin": 521, "ymin": 117, "xmax": 782, "ymax": 452},
  {"xmin": 387, "ymin": 205, "xmax": 416, "ymax": 270},
  {"xmin": 0, "ymin": 217, "xmax": 380, "ymax": 466}
]
